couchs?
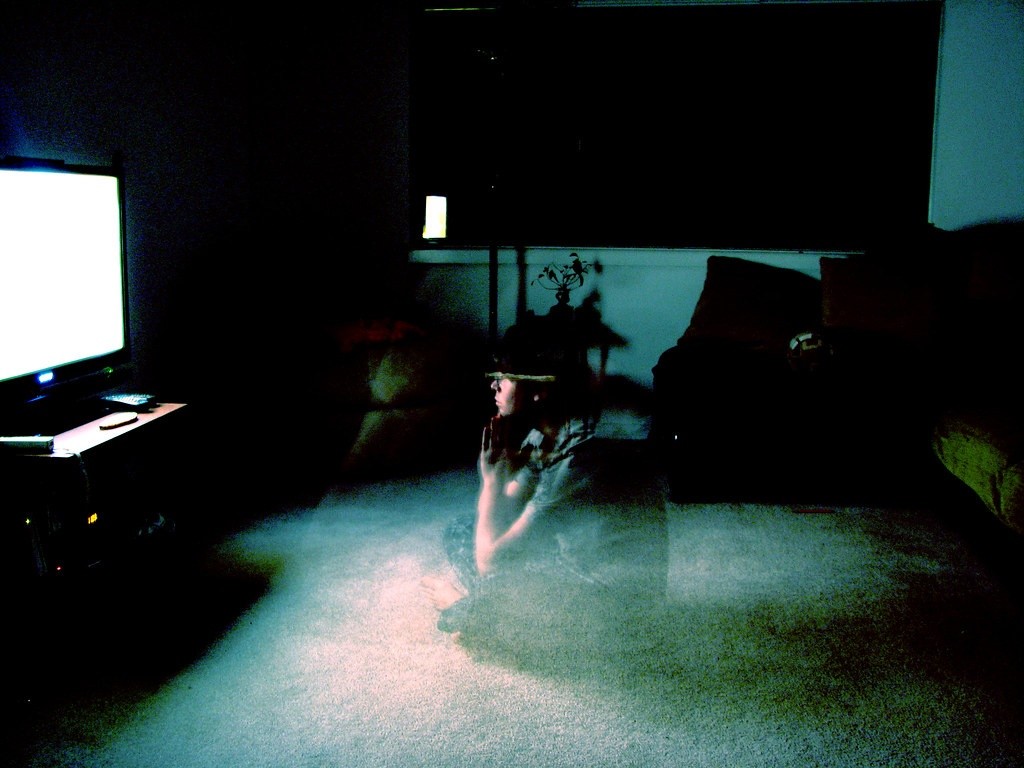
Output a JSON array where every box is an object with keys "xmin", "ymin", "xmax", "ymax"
[{"xmin": 647, "ymin": 217, "xmax": 1024, "ymax": 624}]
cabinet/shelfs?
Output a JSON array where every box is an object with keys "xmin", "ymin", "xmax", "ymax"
[{"xmin": 0, "ymin": 403, "xmax": 189, "ymax": 620}]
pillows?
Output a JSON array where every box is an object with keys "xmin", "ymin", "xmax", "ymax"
[
  {"xmin": 681, "ymin": 256, "xmax": 821, "ymax": 348},
  {"xmin": 821, "ymin": 258, "xmax": 938, "ymax": 338}
]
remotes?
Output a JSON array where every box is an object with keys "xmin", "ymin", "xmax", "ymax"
[
  {"xmin": 0, "ymin": 437, "xmax": 54, "ymax": 452},
  {"xmin": 101, "ymin": 391, "xmax": 156, "ymax": 411}
]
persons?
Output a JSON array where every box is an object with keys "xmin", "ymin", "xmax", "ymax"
[{"xmin": 436, "ymin": 320, "xmax": 668, "ymax": 668}]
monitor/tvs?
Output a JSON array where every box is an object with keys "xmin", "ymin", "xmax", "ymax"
[{"xmin": 0, "ymin": 154, "xmax": 130, "ymax": 396}]
